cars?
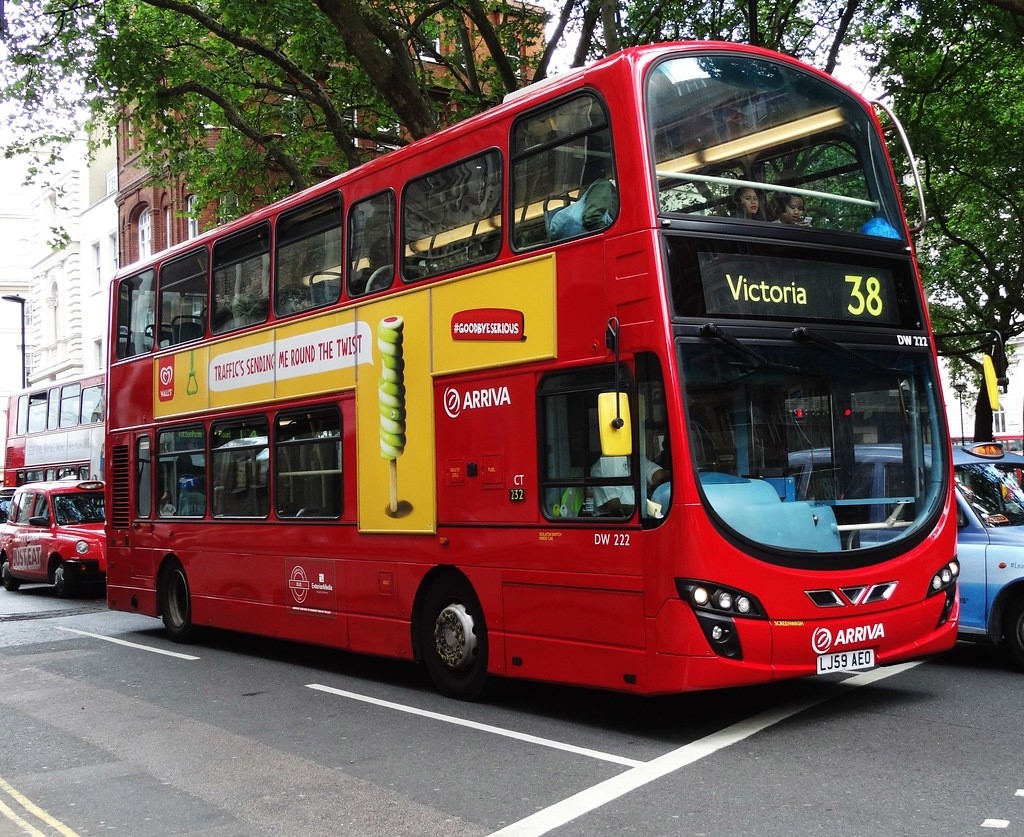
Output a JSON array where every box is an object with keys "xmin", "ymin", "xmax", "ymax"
[
  {"xmin": 787, "ymin": 443, "xmax": 1024, "ymax": 671},
  {"xmin": 0, "ymin": 479, "xmax": 105, "ymax": 599}
]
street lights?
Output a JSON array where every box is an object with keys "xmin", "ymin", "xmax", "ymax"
[{"xmin": 1, "ymin": 294, "xmax": 26, "ymax": 389}]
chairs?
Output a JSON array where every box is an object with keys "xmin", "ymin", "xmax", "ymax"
[
  {"xmin": 115, "ymin": 191, "xmax": 589, "ymax": 363},
  {"xmin": 149, "ymin": 441, "xmax": 344, "ymax": 515}
]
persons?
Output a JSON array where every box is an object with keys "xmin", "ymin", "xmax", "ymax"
[
  {"xmin": 232, "ymin": 275, "xmax": 268, "ymax": 329},
  {"xmin": 580, "ymin": 156, "xmax": 618, "ymax": 232},
  {"xmin": 733, "ymin": 187, "xmax": 813, "ymax": 228},
  {"xmin": 354, "ymin": 236, "xmax": 419, "ymax": 294},
  {"xmin": 590, "ymin": 455, "xmax": 672, "ymax": 518},
  {"xmin": 160, "ymin": 491, "xmax": 175, "ymax": 517}
]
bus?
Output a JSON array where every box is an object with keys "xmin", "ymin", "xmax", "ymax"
[
  {"xmin": 2, "ymin": 369, "xmax": 105, "ymax": 487},
  {"xmin": 104, "ymin": 42, "xmax": 1010, "ymax": 701}
]
